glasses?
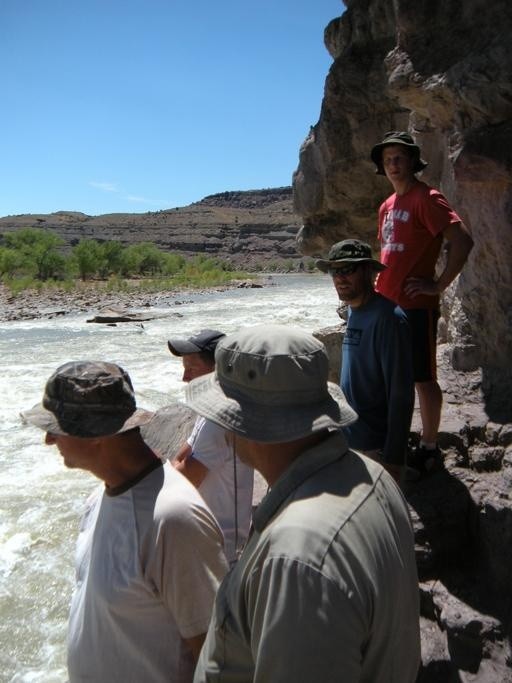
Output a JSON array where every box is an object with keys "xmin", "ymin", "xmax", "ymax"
[{"xmin": 328, "ymin": 266, "xmax": 359, "ymax": 276}]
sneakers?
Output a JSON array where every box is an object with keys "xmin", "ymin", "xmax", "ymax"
[{"xmin": 407, "ymin": 442, "xmax": 444, "ymax": 482}]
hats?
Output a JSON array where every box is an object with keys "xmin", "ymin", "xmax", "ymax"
[
  {"xmin": 315, "ymin": 239, "xmax": 388, "ymax": 273},
  {"xmin": 186, "ymin": 327, "xmax": 359, "ymax": 444},
  {"xmin": 370, "ymin": 131, "xmax": 428, "ymax": 175},
  {"xmin": 19, "ymin": 361, "xmax": 155, "ymax": 438},
  {"xmin": 167, "ymin": 330, "xmax": 224, "ymax": 356}
]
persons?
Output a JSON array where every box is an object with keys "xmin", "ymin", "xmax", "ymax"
[
  {"xmin": 22, "ymin": 358, "xmax": 230, "ymax": 682},
  {"xmin": 185, "ymin": 330, "xmax": 422, "ymax": 683},
  {"xmin": 317, "ymin": 240, "xmax": 415, "ymax": 477},
  {"xmin": 378, "ymin": 129, "xmax": 474, "ymax": 453},
  {"xmin": 169, "ymin": 326, "xmax": 254, "ymax": 568}
]
